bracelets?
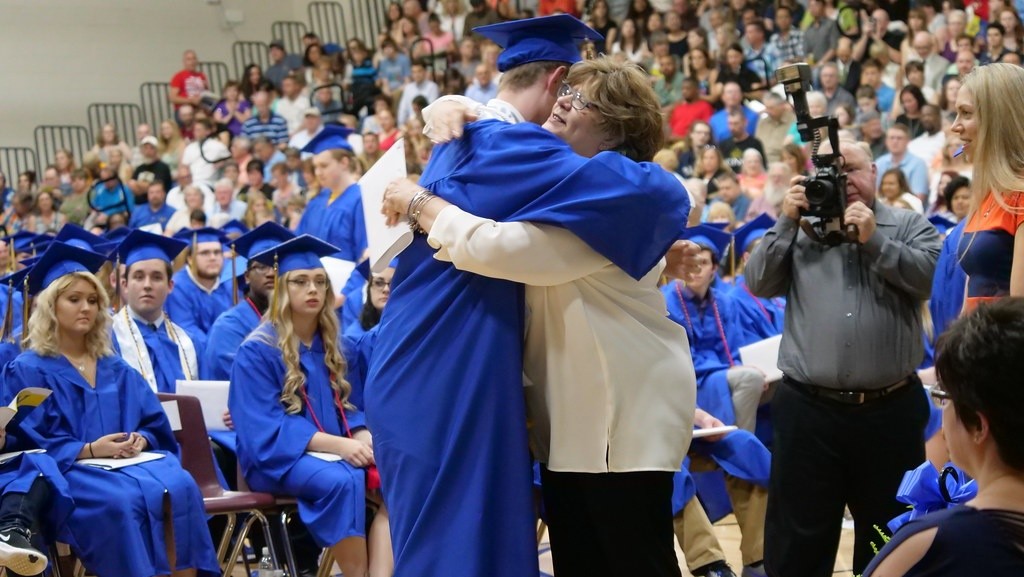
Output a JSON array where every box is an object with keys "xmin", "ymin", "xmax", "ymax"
[
  {"xmin": 407, "ymin": 189, "xmax": 427, "ymax": 220},
  {"xmin": 409, "ymin": 192, "xmax": 433, "ymax": 233},
  {"xmin": 412, "ymin": 191, "xmax": 435, "ymax": 234},
  {"xmin": 89, "ymin": 443, "xmax": 94, "ymax": 458}
]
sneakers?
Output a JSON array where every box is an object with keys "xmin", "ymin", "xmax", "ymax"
[
  {"xmin": 694, "ymin": 561, "xmax": 737, "ymax": 577},
  {"xmin": 0, "ymin": 526, "xmax": 48, "ymax": 577},
  {"xmin": 741, "ymin": 563, "xmax": 767, "ymax": 577}
]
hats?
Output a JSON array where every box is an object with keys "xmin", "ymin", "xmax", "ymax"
[
  {"xmin": 141, "ymin": 136, "xmax": 159, "ymax": 149},
  {"xmin": 355, "ymin": 256, "xmax": 402, "ymax": 281},
  {"xmin": 674, "ymin": 225, "xmax": 734, "ymax": 284},
  {"xmin": 700, "ymin": 221, "xmax": 729, "ymax": 230},
  {"xmin": 300, "ymin": 125, "xmax": 355, "ymax": 155},
  {"xmin": 0, "ymin": 219, "xmax": 342, "ymax": 350},
  {"xmin": 953, "ymin": 145, "xmax": 964, "ymax": 157},
  {"xmin": 929, "ymin": 215, "xmax": 956, "ymax": 235},
  {"xmin": 472, "ymin": 13, "xmax": 604, "ymax": 73},
  {"xmin": 732, "ymin": 212, "xmax": 776, "ymax": 258}
]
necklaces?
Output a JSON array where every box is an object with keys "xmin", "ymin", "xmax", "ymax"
[{"xmin": 79, "ymin": 365, "xmax": 85, "ymax": 371}]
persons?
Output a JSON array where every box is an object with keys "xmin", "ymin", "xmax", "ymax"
[
  {"xmin": 0, "ymin": 0, "xmax": 1024, "ymax": 577},
  {"xmin": 950, "ymin": 62, "xmax": 1024, "ymax": 309},
  {"xmin": 873, "ymin": 296, "xmax": 1024, "ymax": 577},
  {"xmin": 362, "ymin": 14, "xmax": 702, "ymax": 577},
  {"xmin": 381, "ymin": 55, "xmax": 697, "ymax": 577}
]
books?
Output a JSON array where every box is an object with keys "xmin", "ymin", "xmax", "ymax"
[{"xmin": 360, "ymin": 139, "xmax": 414, "ymax": 274}]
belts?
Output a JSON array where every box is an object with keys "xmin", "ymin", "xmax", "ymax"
[{"xmin": 782, "ymin": 372, "xmax": 917, "ymax": 404}]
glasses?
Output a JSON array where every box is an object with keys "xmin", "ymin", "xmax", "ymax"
[
  {"xmin": 557, "ymin": 80, "xmax": 600, "ymax": 111},
  {"xmin": 370, "ymin": 277, "xmax": 393, "ymax": 289},
  {"xmin": 930, "ymin": 382, "xmax": 952, "ymax": 410},
  {"xmin": 286, "ymin": 277, "xmax": 330, "ymax": 290},
  {"xmin": 249, "ymin": 263, "xmax": 276, "ymax": 274}
]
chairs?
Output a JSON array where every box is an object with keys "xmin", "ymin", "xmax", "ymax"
[{"xmin": 48, "ymin": 394, "xmax": 379, "ymax": 577}]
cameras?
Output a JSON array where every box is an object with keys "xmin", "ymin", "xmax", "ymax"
[{"xmin": 776, "ymin": 62, "xmax": 847, "ymax": 220}]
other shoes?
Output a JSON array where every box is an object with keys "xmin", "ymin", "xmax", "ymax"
[
  {"xmin": 298, "ymin": 563, "xmax": 320, "ymax": 577},
  {"xmin": 232, "ymin": 535, "xmax": 256, "ymax": 563}
]
